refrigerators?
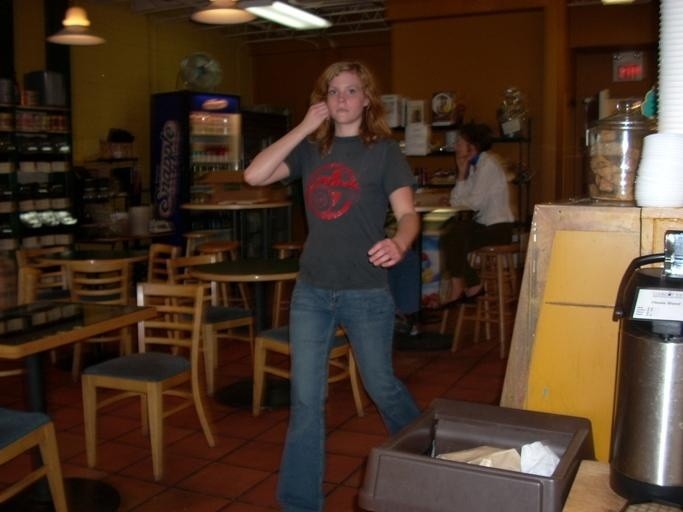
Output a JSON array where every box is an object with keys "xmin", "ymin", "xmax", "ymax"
[{"xmin": 149, "ymin": 91, "xmax": 244, "ymax": 247}]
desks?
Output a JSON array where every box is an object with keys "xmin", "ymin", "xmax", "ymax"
[
  {"xmin": 190, "ymin": 258, "xmax": 296, "ymax": 410},
  {"xmin": 559, "ymin": 460, "xmax": 683, "ymax": 511},
  {"xmin": 186, "ymin": 199, "xmax": 291, "ymax": 255}
]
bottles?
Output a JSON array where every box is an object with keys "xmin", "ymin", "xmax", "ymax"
[{"xmin": 583, "ymin": 99, "xmax": 650, "ymax": 207}]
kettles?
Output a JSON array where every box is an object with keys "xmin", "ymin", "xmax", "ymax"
[{"xmin": 602, "ymin": 228, "xmax": 683, "ymax": 503}]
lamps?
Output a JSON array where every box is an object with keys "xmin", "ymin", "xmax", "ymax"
[
  {"xmin": 189, "ymin": 1, "xmax": 258, "ymax": 24},
  {"xmin": 46, "ymin": 0, "xmax": 107, "ymax": 46},
  {"xmin": 235, "ymin": 1, "xmax": 332, "ymax": 33}
]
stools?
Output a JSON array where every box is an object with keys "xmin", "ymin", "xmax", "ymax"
[{"xmin": 449, "ymin": 243, "xmax": 519, "ymax": 359}]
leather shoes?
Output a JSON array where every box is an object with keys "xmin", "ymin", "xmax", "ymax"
[{"xmin": 442, "ymin": 287, "xmax": 485, "ymax": 309}]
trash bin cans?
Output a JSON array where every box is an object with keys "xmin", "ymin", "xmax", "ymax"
[{"xmin": 357, "ymin": 396, "xmax": 598, "ymax": 512}]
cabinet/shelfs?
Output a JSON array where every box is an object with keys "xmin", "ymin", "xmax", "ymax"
[
  {"xmin": 393, "ymin": 122, "xmax": 462, "ymax": 192},
  {"xmin": 0, "ymin": 104, "xmax": 70, "ymax": 240},
  {"xmin": 470, "ymin": 133, "xmax": 530, "ymax": 232}
]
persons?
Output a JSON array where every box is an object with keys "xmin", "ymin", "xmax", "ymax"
[
  {"xmin": 244, "ymin": 60, "xmax": 423, "ymax": 512},
  {"xmin": 437, "ymin": 122, "xmax": 514, "ymax": 308}
]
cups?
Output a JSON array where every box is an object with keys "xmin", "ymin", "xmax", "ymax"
[{"xmin": 656, "ymin": 0, "xmax": 683, "ymax": 134}]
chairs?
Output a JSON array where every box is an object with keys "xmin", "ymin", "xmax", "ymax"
[
  {"xmin": 2, "ymin": 230, "xmax": 309, "ymax": 381},
  {"xmin": 83, "ymin": 279, "xmax": 216, "ymax": 482},
  {"xmin": 2, "ymin": 301, "xmax": 159, "ymax": 512},
  {"xmin": 166, "ymin": 256, "xmax": 257, "ymax": 395},
  {"xmin": 252, "ymin": 321, "xmax": 366, "ymax": 423},
  {"xmin": 2, "ymin": 407, "xmax": 68, "ymax": 510}
]
